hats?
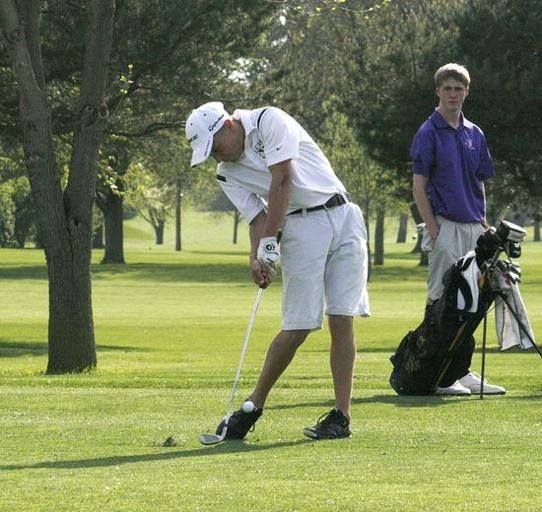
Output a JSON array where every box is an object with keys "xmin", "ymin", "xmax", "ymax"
[{"xmin": 184, "ymin": 101, "xmax": 228, "ymax": 168}]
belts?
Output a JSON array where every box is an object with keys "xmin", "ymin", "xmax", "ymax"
[{"xmin": 284, "ymin": 194, "xmax": 345, "ymax": 216}]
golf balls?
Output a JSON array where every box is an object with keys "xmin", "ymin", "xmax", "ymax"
[{"xmin": 242, "ymin": 401, "xmax": 255, "ymax": 413}]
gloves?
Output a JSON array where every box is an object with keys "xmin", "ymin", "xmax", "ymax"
[{"xmin": 259, "ymin": 231, "xmax": 282, "ymax": 276}]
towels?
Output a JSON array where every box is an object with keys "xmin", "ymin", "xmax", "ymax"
[{"xmin": 456, "ymin": 250, "xmax": 536, "ymax": 352}]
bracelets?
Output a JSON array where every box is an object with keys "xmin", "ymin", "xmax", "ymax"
[{"xmin": 430, "ymin": 232, "xmax": 438, "ymax": 240}]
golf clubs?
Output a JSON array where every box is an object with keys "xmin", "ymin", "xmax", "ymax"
[{"xmin": 198, "ymin": 228, "xmax": 283, "ymax": 445}]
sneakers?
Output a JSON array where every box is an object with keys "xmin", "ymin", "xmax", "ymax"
[
  {"xmin": 435, "ymin": 371, "xmax": 506, "ymax": 395},
  {"xmin": 303, "ymin": 408, "xmax": 352, "ymax": 439},
  {"xmin": 215, "ymin": 397, "xmax": 263, "ymax": 440}
]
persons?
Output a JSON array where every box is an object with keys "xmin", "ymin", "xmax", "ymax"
[
  {"xmin": 181, "ymin": 101, "xmax": 372, "ymax": 440},
  {"xmin": 409, "ymin": 61, "xmax": 508, "ymax": 399}
]
